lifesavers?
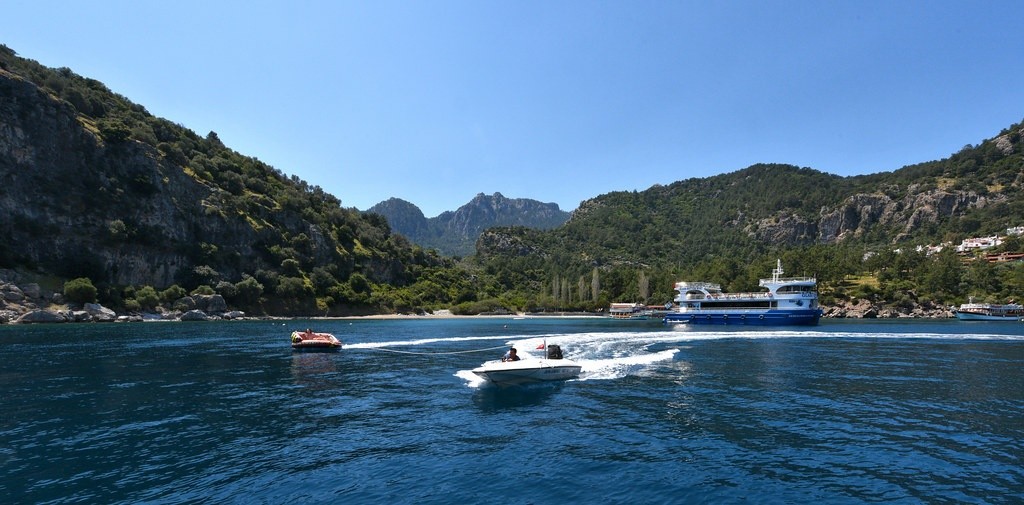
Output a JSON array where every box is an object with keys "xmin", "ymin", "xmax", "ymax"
[
  {"xmin": 706, "ymin": 314, "xmax": 711, "ymax": 320},
  {"xmin": 707, "ymin": 295, "xmax": 711, "ymax": 299},
  {"xmin": 690, "ymin": 314, "xmax": 695, "ymax": 319},
  {"xmin": 741, "ymin": 315, "xmax": 745, "ymax": 320},
  {"xmin": 758, "ymin": 314, "xmax": 764, "ymax": 320},
  {"xmin": 723, "ymin": 314, "xmax": 728, "ymax": 320},
  {"xmin": 768, "ymin": 293, "xmax": 773, "ymax": 298}
]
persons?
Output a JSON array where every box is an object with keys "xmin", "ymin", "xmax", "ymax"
[
  {"xmin": 302, "ymin": 328, "xmax": 316, "ymax": 340},
  {"xmin": 508, "ymin": 348, "xmax": 521, "ymax": 361}
]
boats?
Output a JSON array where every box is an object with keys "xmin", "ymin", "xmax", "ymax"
[
  {"xmin": 662, "ymin": 258, "xmax": 823, "ymax": 326},
  {"xmin": 956, "ymin": 310, "xmax": 1024, "ymax": 321},
  {"xmin": 609, "ymin": 302, "xmax": 650, "ymax": 320},
  {"xmin": 291, "ymin": 330, "xmax": 343, "ymax": 350},
  {"xmin": 471, "ymin": 338, "xmax": 583, "ymax": 383}
]
